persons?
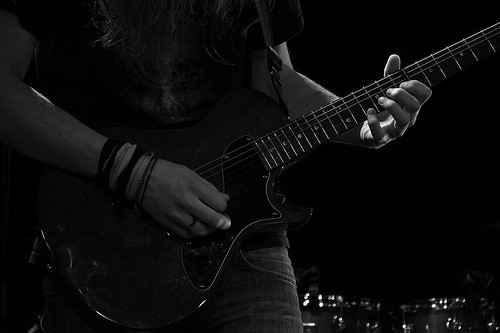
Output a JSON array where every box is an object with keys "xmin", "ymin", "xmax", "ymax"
[{"xmin": 0, "ymin": 0, "xmax": 435, "ymax": 333}]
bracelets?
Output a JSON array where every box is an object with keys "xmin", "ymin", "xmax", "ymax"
[{"xmin": 95, "ymin": 136, "xmax": 164, "ymax": 211}]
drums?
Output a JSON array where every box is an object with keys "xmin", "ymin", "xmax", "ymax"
[
  {"xmin": 296, "ymin": 288, "xmax": 381, "ymax": 333},
  {"xmin": 392, "ymin": 297, "xmax": 498, "ymax": 333}
]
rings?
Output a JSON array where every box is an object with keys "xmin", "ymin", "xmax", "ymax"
[{"xmin": 187, "ymin": 219, "xmax": 198, "ymax": 230}]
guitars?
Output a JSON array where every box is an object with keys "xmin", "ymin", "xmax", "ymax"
[{"xmin": 35, "ymin": 19, "xmax": 500, "ymax": 330}]
